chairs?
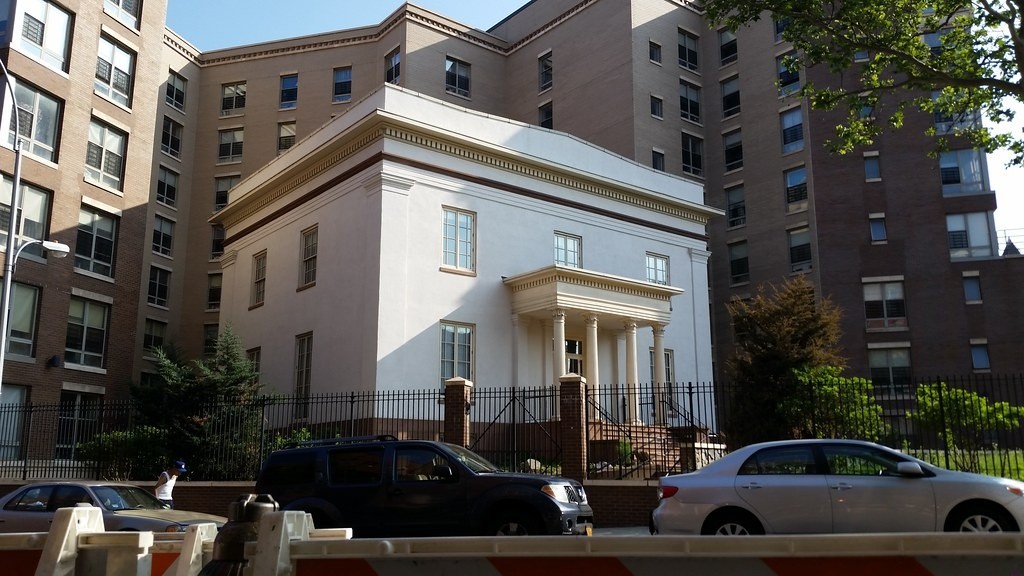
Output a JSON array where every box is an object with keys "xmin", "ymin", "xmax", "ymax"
[{"xmin": 416, "ymin": 475, "xmax": 428, "ymax": 480}]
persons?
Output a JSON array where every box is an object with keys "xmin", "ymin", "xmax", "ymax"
[{"xmin": 151, "ymin": 461, "xmax": 188, "ymax": 509}]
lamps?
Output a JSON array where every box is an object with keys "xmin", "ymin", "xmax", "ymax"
[{"xmin": 47, "ymin": 355, "xmax": 60, "ymax": 367}]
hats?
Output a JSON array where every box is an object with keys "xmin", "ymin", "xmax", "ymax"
[{"xmin": 174, "ymin": 461, "xmax": 187, "ymax": 472}]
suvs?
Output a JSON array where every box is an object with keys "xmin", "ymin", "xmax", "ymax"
[{"xmin": 253, "ymin": 433, "xmax": 595, "ymax": 539}]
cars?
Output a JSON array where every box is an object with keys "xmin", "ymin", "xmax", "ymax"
[
  {"xmin": 0, "ymin": 479, "xmax": 228, "ymax": 535},
  {"xmin": 653, "ymin": 439, "xmax": 1024, "ymax": 536}
]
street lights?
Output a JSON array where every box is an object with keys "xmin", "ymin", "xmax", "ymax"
[{"xmin": 0, "ymin": 241, "xmax": 71, "ymax": 399}]
